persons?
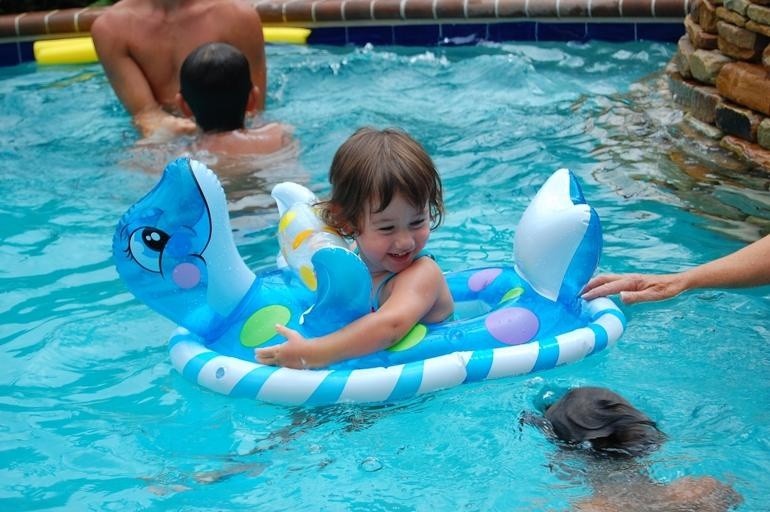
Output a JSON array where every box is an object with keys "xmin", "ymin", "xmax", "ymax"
[
  {"xmin": 180, "ymin": 42, "xmax": 294, "ymax": 181},
  {"xmin": 579, "ymin": 234, "xmax": 769, "ymax": 305},
  {"xmin": 254, "ymin": 127, "xmax": 458, "ymax": 371},
  {"xmin": 90, "ymin": 0, "xmax": 268, "ymax": 139},
  {"xmin": 543, "ymin": 385, "xmax": 745, "ymax": 510}
]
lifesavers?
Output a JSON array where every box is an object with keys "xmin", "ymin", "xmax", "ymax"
[{"xmin": 112, "ymin": 157, "xmax": 627, "ymax": 408}]
